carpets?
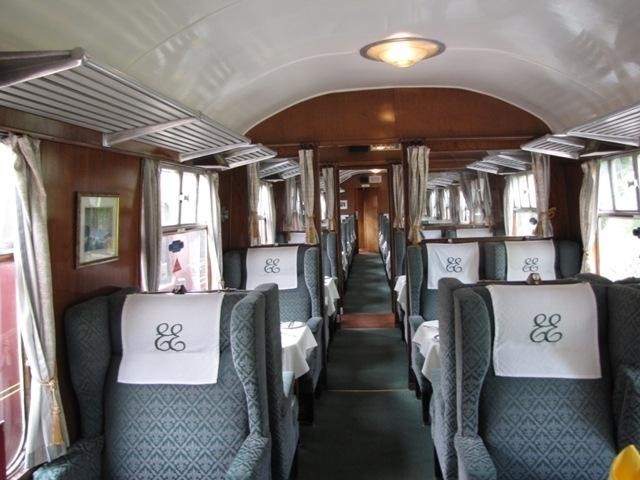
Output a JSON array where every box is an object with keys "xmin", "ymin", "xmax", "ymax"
[{"xmin": 297, "ymin": 252, "xmax": 435, "ymax": 479}]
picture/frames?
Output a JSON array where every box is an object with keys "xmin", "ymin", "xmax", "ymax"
[{"xmin": 75, "ymin": 192, "xmax": 122, "ymax": 269}]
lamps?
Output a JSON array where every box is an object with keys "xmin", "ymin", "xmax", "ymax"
[{"xmin": 358, "ymin": 30, "xmax": 446, "ymax": 70}]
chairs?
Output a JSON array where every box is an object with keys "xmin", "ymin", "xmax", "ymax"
[
  {"xmin": 378, "ymin": 213, "xmax": 639, "ymax": 480},
  {"xmin": 30, "ymin": 214, "xmax": 357, "ymax": 479}
]
